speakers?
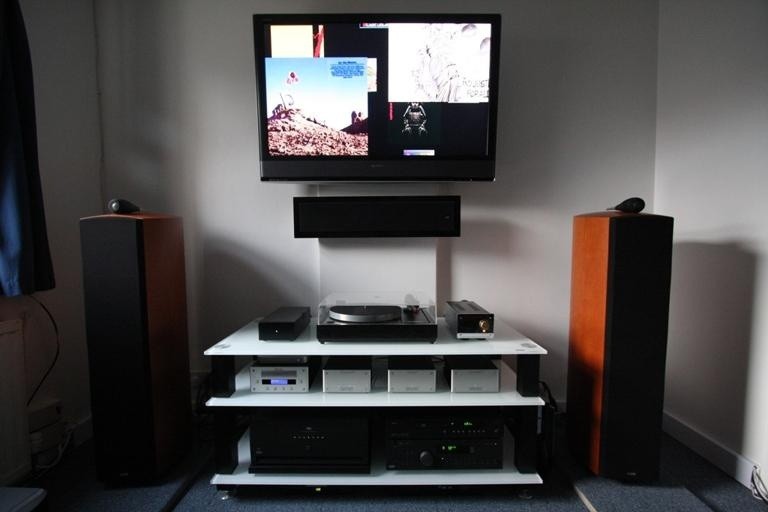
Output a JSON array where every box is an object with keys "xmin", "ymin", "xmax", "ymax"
[
  {"xmin": 561, "ymin": 209, "xmax": 675, "ymax": 473},
  {"xmin": 79, "ymin": 210, "xmax": 193, "ymax": 486}
]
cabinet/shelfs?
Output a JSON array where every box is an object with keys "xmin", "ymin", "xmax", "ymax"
[{"xmin": 203, "ymin": 315, "xmax": 548, "ymax": 500}]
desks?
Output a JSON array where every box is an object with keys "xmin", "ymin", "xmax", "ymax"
[{"xmin": 0, "ymin": 486, "xmax": 47, "ymax": 512}]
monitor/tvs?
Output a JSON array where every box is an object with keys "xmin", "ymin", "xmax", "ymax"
[{"xmin": 252, "ymin": 11, "xmax": 502, "ymax": 183}]
